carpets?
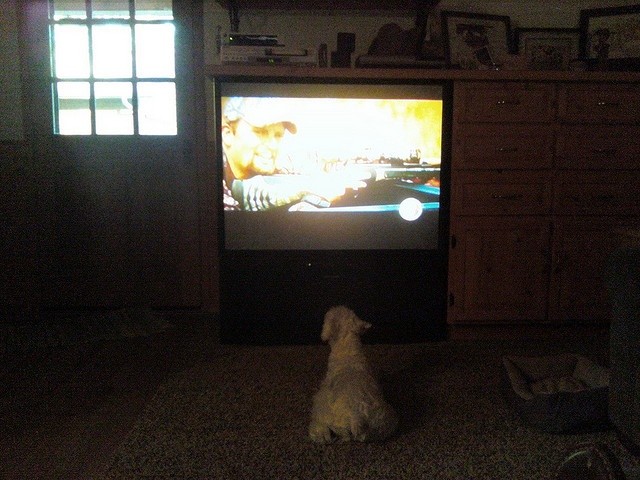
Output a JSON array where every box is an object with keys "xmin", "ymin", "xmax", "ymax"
[{"xmin": 98, "ymin": 342, "xmax": 637, "ymax": 479}]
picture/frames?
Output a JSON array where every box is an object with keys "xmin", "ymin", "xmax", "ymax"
[
  {"xmin": 441, "ymin": 11, "xmax": 514, "ymax": 68},
  {"xmin": 580, "ymin": 5, "xmax": 640, "ymax": 68},
  {"xmin": 515, "ymin": 26, "xmax": 585, "ymax": 68}
]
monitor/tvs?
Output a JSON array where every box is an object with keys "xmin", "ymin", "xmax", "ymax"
[{"xmin": 217, "ymin": 80, "xmax": 450, "ymax": 343}]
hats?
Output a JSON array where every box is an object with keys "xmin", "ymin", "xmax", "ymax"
[{"xmin": 222, "ymin": 97, "xmax": 299, "ymax": 134}]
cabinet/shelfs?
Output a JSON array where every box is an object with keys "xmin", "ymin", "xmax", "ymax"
[{"xmin": 449, "ymin": 69, "xmax": 639, "ymax": 327}]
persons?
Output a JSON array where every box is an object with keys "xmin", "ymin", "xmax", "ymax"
[{"xmin": 220, "ymin": 96, "xmax": 369, "ymax": 212}]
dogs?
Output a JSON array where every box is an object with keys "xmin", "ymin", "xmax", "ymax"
[{"xmin": 310, "ymin": 306, "xmax": 399, "ymax": 444}]
